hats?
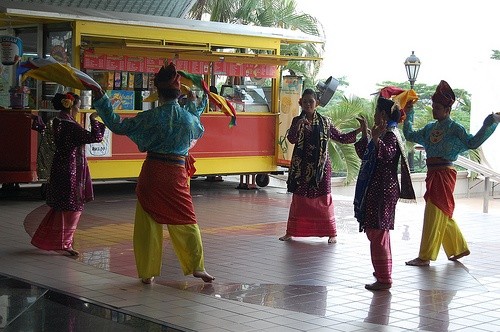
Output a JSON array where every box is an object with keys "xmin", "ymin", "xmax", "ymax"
[
  {"xmin": 377, "ymin": 83, "xmax": 418, "ymax": 122},
  {"xmin": 153, "ymin": 63, "xmax": 183, "ymax": 96},
  {"xmin": 432, "ymin": 80, "xmax": 456, "ymax": 105}
]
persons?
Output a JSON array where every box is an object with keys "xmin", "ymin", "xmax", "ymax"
[
  {"xmin": 93, "ymin": 73, "xmax": 218, "ymax": 285},
  {"xmin": 403, "ymin": 93, "xmax": 500, "ymax": 265},
  {"xmin": 25, "ymin": 92, "xmax": 104, "ymax": 255},
  {"xmin": 354, "ymin": 99, "xmax": 401, "ymax": 290},
  {"xmin": 278, "ymin": 89, "xmax": 372, "ymax": 243}
]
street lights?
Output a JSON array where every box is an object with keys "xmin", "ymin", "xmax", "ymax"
[{"xmin": 404, "ymin": 50, "xmax": 421, "ymax": 173}]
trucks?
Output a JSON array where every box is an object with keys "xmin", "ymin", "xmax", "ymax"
[{"xmin": 0, "ymin": 0, "xmax": 324, "ymax": 201}]
